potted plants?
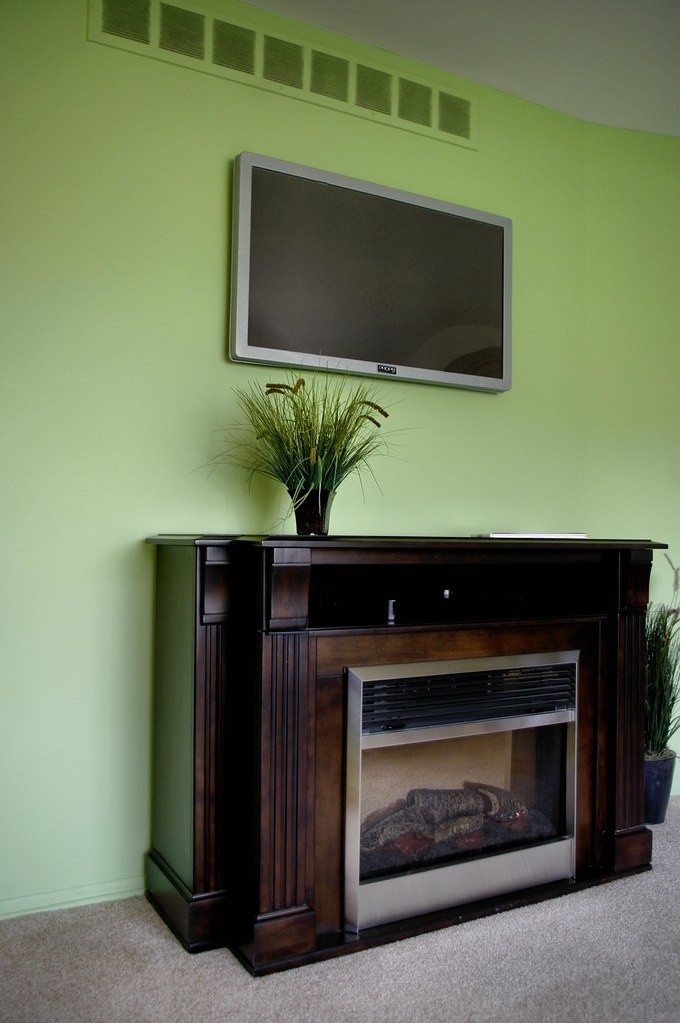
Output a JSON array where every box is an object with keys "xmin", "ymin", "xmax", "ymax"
[
  {"xmin": 642, "ymin": 552, "xmax": 680, "ymax": 824},
  {"xmin": 189, "ymin": 351, "xmax": 419, "ymax": 537}
]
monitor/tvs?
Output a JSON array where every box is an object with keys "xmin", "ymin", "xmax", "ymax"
[{"xmin": 233, "ymin": 150, "xmax": 515, "ymax": 394}]
